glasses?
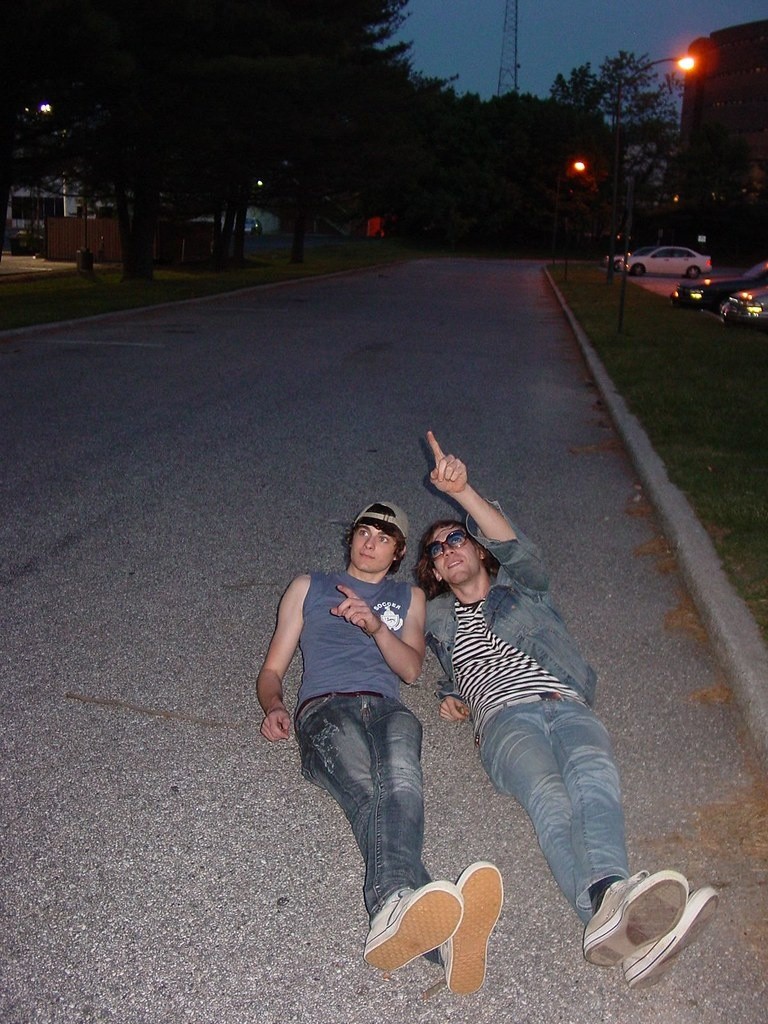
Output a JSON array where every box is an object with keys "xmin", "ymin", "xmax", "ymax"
[{"xmin": 428, "ymin": 530, "xmax": 470, "ymax": 559}]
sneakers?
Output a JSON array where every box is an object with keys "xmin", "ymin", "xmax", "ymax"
[
  {"xmin": 582, "ymin": 869, "xmax": 689, "ymax": 965},
  {"xmin": 363, "ymin": 880, "xmax": 465, "ymax": 971},
  {"xmin": 438, "ymin": 861, "xmax": 504, "ymax": 995},
  {"xmin": 623, "ymin": 886, "xmax": 720, "ymax": 989}
]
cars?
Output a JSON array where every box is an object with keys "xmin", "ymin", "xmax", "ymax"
[
  {"xmin": 604, "ymin": 246, "xmax": 655, "ymax": 273},
  {"xmin": 720, "ymin": 284, "xmax": 767, "ymax": 331},
  {"xmin": 671, "ymin": 260, "xmax": 768, "ymax": 311},
  {"xmin": 618, "ymin": 245, "xmax": 714, "ymax": 280},
  {"xmin": 232, "ymin": 217, "xmax": 263, "ymax": 235}
]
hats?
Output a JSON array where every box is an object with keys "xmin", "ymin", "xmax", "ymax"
[{"xmin": 353, "ymin": 502, "xmax": 410, "ymax": 545}]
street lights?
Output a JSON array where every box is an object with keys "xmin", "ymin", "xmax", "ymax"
[
  {"xmin": 616, "ymin": 55, "xmax": 695, "ymax": 332},
  {"xmin": 551, "ymin": 159, "xmax": 586, "ymax": 264}
]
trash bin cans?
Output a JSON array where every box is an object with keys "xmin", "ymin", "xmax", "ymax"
[
  {"xmin": 9, "ymin": 230, "xmax": 41, "ymax": 257},
  {"xmin": 170, "ymin": 222, "xmax": 207, "ymax": 267}
]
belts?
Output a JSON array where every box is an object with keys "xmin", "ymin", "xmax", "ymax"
[
  {"xmin": 494, "ymin": 692, "xmax": 570, "ymax": 715},
  {"xmin": 295, "ymin": 691, "xmax": 389, "ymax": 720}
]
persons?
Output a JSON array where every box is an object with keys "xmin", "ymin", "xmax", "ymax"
[
  {"xmin": 259, "ymin": 498, "xmax": 501, "ymax": 990},
  {"xmin": 410, "ymin": 432, "xmax": 719, "ymax": 988}
]
